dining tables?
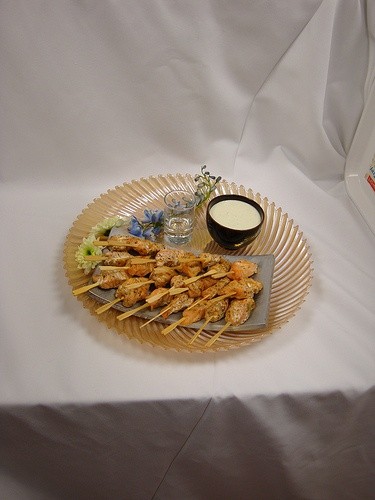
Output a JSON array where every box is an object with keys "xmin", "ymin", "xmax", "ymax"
[{"xmin": 0, "ymin": 0, "xmax": 375, "ymax": 500}]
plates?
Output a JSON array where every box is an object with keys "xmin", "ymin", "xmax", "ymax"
[
  {"xmin": 62, "ymin": 176, "xmax": 312, "ymax": 355},
  {"xmin": 88, "ymin": 227, "xmax": 274, "ymax": 331}
]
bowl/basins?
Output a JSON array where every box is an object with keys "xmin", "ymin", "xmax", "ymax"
[{"xmin": 206, "ymin": 194, "xmax": 264, "ymax": 250}]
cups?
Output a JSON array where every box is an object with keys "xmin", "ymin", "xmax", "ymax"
[{"xmin": 163, "ymin": 190, "xmax": 196, "ymax": 246}]
